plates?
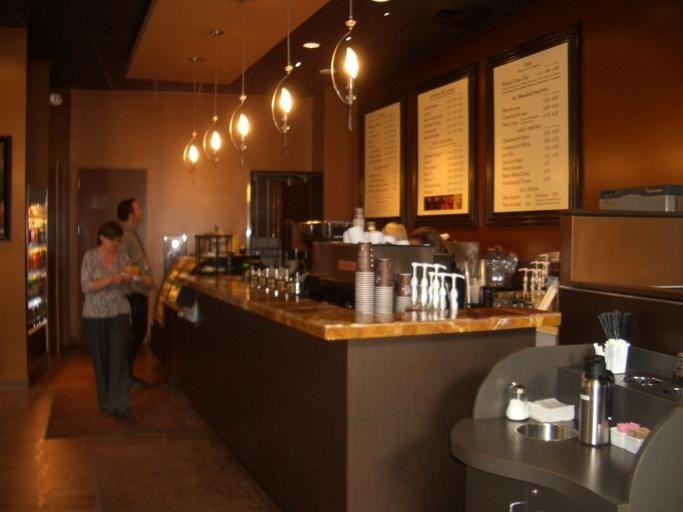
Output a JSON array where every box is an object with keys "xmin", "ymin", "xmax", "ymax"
[{"xmin": 610, "ymin": 423, "xmax": 650, "ymax": 455}]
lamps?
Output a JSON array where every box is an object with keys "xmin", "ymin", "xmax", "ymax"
[{"xmin": 180, "ymin": 1, "xmax": 362, "ymax": 177}]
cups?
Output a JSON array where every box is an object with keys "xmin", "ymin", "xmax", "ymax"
[{"xmin": 354, "ymin": 242, "xmax": 413, "ymax": 316}]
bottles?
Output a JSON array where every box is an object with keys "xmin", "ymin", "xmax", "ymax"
[
  {"xmin": 579, "ymin": 355, "xmax": 614, "ymax": 448},
  {"xmin": 249, "ymin": 264, "xmax": 301, "ymax": 296},
  {"xmin": 506, "ymin": 380, "xmax": 531, "ymax": 422}
]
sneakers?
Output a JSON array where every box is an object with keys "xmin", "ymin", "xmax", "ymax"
[{"xmin": 99, "ymin": 374, "xmax": 146, "ymax": 420}]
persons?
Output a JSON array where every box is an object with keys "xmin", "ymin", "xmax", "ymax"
[
  {"xmin": 77, "ymin": 221, "xmax": 139, "ymax": 419},
  {"xmin": 113, "ymin": 196, "xmax": 151, "ymax": 388}
]
